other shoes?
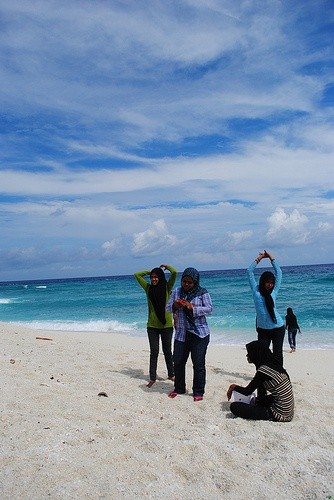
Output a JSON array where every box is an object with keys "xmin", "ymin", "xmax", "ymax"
[
  {"xmin": 169, "ymin": 391, "xmax": 178, "ymax": 398},
  {"xmin": 194, "ymin": 396, "xmax": 203, "ymax": 401}
]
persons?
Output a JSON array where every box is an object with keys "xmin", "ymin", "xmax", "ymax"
[
  {"xmin": 285, "ymin": 307, "xmax": 301, "ymax": 353},
  {"xmin": 247, "ymin": 250, "xmax": 285, "ymax": 397},
  {"xmin": 227, "ymin": 340, "xmax": 294, "ymax": 422},
  {"xmin": 134, "ymin": 264, "xmax": 178, "ymax": 387},
  {"xmin": 169, "ymin": 267, "xmax": 213, "ymax": 402}
]
